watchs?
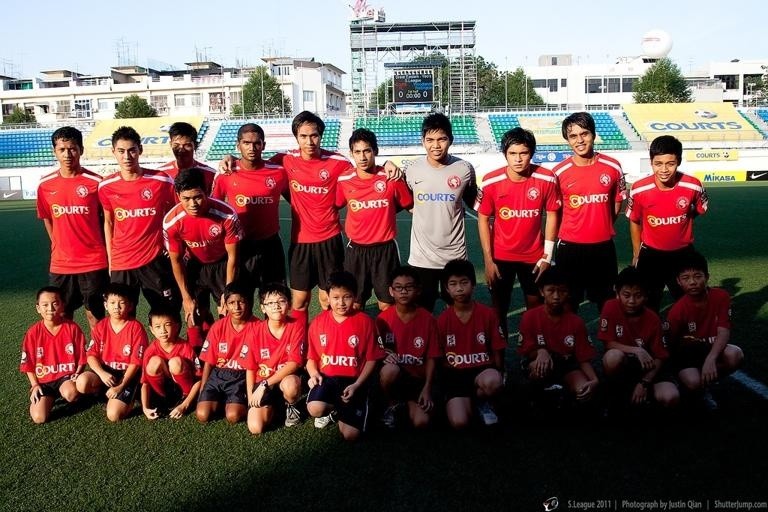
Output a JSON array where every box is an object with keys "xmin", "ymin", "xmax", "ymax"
[
  {"xmin": 640, "ymin": 380, "xmax": 652, "ymax": 388},
  {"xmin": 261, "ymin": 380, "xmax": 271, "ymax": 390}
]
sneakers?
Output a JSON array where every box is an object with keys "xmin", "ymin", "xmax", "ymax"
[
  {"xmin": 703, "ymin": 389, "xmax": 720, "ymax": 411},
  {"xmin": 285, "ymin": 402, "xmax": 300, "ymax": 428},
  {"xmin": 478, "ymin": 398, "xmax": 499, "ymax": 426},
  {"xmin": 313, "ymin": 413, "xmax": 336, "ymax": 428},
  {"xmin": 380, "ymin": 402, "xmax": 401, "ymax": 427}
]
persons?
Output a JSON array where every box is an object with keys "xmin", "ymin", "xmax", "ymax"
[
  {"xmin": 307, "ymin": 271, "xmax": 384, "ymax": 440},
  {"xmin": 625, "ymin": 135, "xmax": 709, "ymax": 320},
  {"xmin": 598, "ymin": 266, "xmax": 678, "ymax": 404},
  {"xmin": 76, "ymin": 282, "xmax": 148, "ymax": 422},
  {"xmin": 552, "ymin": 111, "xmax": 630, "ymax": 321},
  {"xmin": 197, "ymin": 281, "xmax": 261, "ymax": 423},
  {"xmin": 247, "ymin": 282, "xmax": 305, "ymax": 433},
  {"xmin": 436, "ymin": 258, "xmax": 508, "ymax": 427},
  {"xmin": 518, "ymin": 269, "xmax": 598, "ymax": 401},
  {"xmin": 370, "ymin": 266, "xmax": 441, "ymax": 426},
  {"xmin": 163, "ymin": 168, "xmax": 243, "ymax": 366},
  {"xmin": 21, "ymin": 286, "xmax": 87, "ymax": 423},
  {"xmin": 156, "ymin": 122, "xmax": 218, "ymax": 198},
  {"xmin": 478, "ymin": 127, "xmax": 562, "ymax": 342},
  {"xmin": 405, "ymin": 112, "xmax": 482, "ymax": 313},
  {"xmin": 37, "ymin": 126, "xmax": 104, "ymax": 334},
  {"xmin": 210, "ymin": 124, "xmax": 291, "ymax": 317},
  {"xmin": 140, "ymin": 304, "xmax": 203, "ymax": 419},
  {"xmin": 98, "ymin": 127, "xmax": 183, "ymax": 338},
  {"xmin": 664, "ymin": 250, "xmax": 744, "ymax": 409},
  {"xmin": 218, "ymin": 110, "xmax": 405, "ymax": 319},
  {"xmin": 335, "ymin": 128, "xmax": 414, "ymax": 312}
]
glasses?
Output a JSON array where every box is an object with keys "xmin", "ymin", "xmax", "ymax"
[
  {"xmin": 390, "ymin": 283, "xmax": 418, "ymax": 292},
  {"xmin": 262, "ymin": 299, "xmax": 289, "ymax": 306}
]
